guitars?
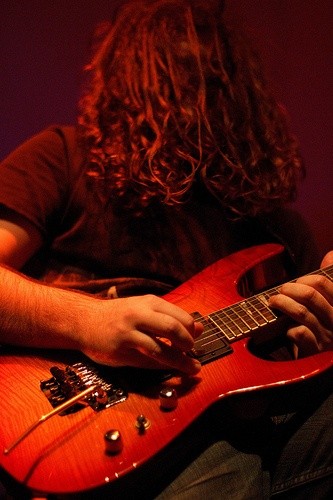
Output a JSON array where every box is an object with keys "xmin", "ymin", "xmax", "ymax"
[{"xmin": 0, "ymin": 243, "xmax": 333, "ymax": 493}]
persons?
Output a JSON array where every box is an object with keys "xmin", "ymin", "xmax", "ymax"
[{"xmin": 0, "ymin": 1, "xmax": 332, "ymax": 500}]
